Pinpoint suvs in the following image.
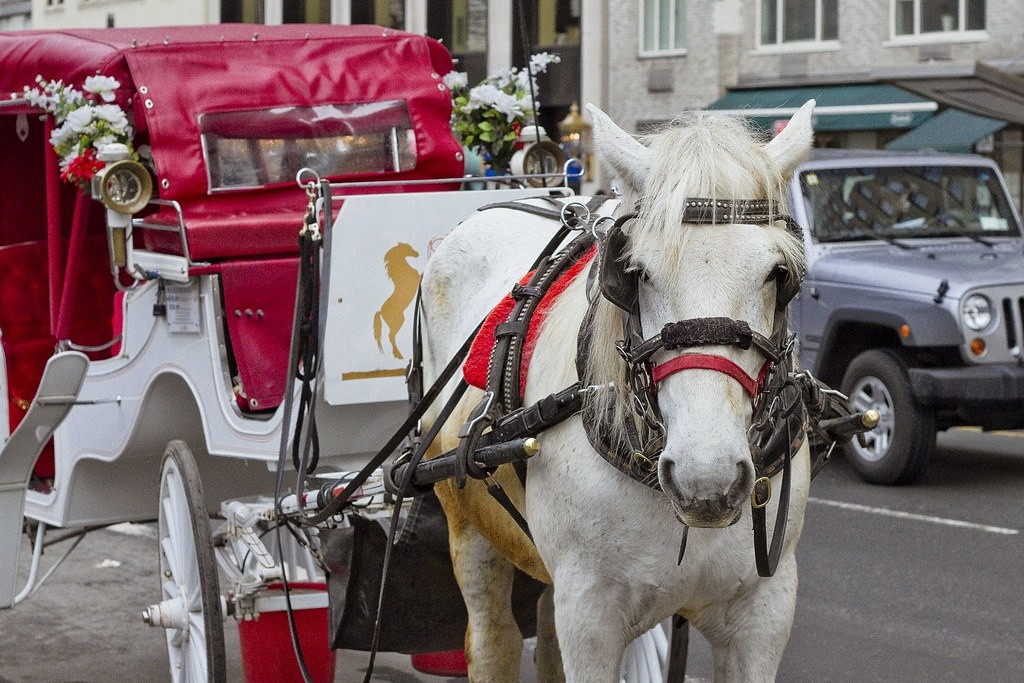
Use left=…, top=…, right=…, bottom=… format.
left=762, top=146, right=1024, bottom=488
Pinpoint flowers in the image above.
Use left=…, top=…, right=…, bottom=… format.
left=442, top=49, right=560, bottom=172
left=10, top=72, right=135, bottom=186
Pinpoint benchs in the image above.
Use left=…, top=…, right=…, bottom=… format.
left=0, top=234, right=115, bottom=436
left=142, top=189, right=448, bottom=411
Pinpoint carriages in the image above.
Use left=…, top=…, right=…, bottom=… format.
left=0, top=23, right=819, bottom=683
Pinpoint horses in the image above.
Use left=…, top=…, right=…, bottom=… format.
left=408, top=99, right=815, bottom=683
